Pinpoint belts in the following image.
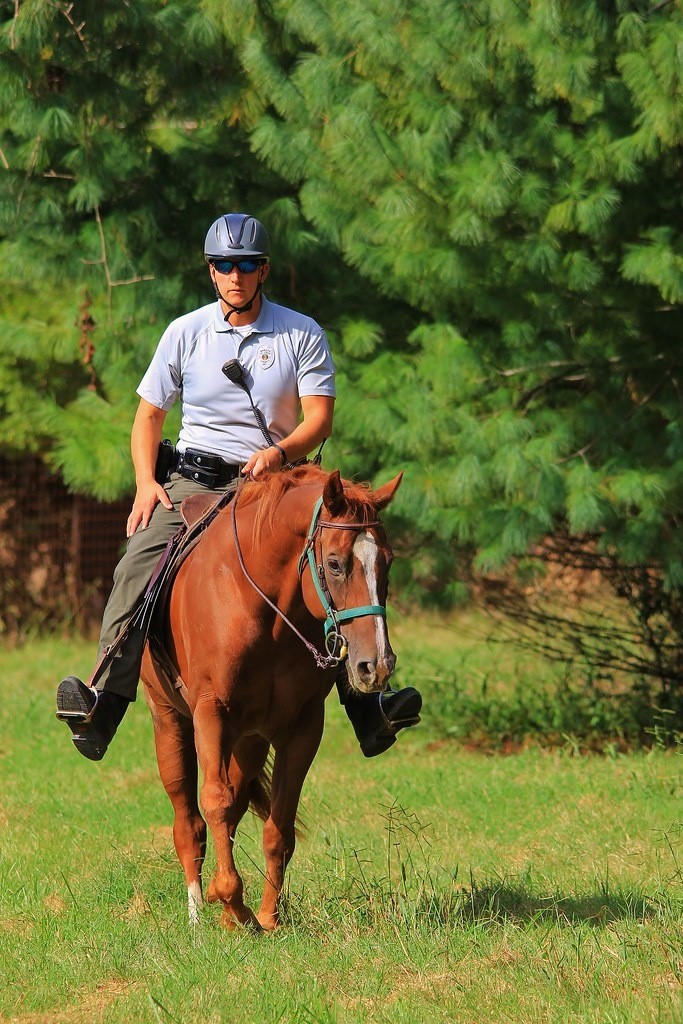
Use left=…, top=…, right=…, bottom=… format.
left=229, top=464, right=248, bottom=480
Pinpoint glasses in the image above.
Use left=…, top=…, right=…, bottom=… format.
left=208, top=258, right=267, bottom=275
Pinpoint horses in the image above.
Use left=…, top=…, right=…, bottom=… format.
left=140, top=461, right=406, bottom=938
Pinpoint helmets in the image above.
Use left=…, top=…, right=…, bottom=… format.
left=203, top=213, right=270, bottom=263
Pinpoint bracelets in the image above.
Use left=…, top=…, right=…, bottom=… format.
left=271, top=444, right=287, bottom=468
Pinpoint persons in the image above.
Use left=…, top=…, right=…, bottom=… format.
left=56, top=214, right=424, bottom=762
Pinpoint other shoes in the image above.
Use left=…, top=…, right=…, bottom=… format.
left=344, top=686, right=422, bottom=758
left=56, top=675, right=130, bottom=761
